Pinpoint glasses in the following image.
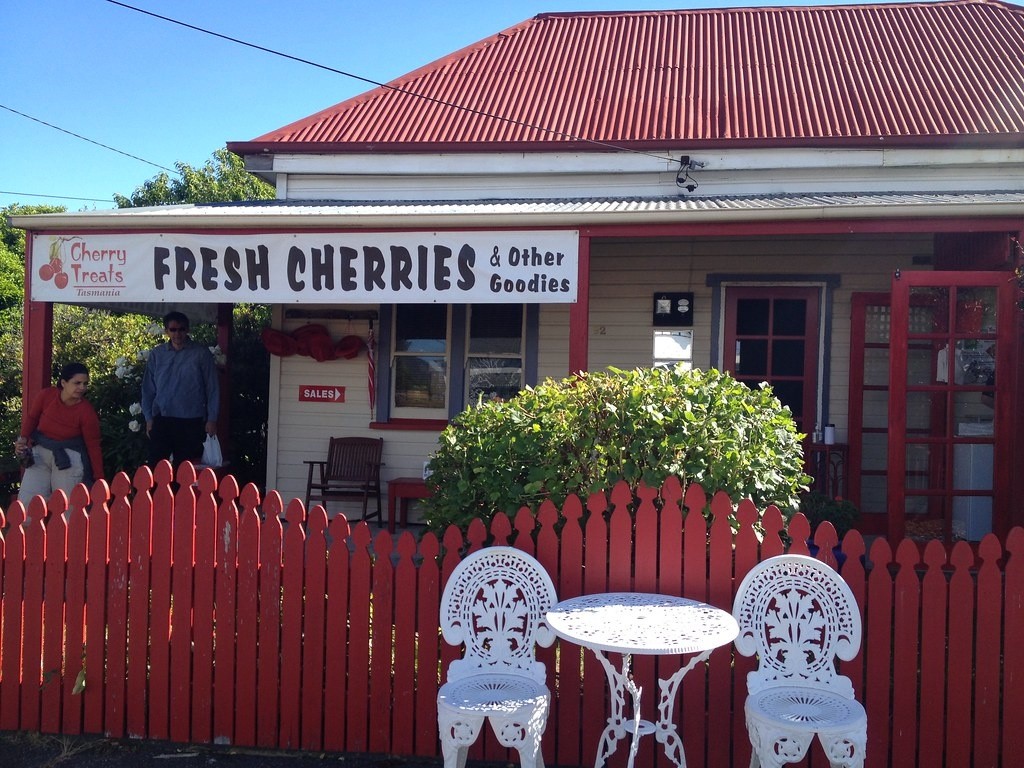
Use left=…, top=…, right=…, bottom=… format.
left=167, top=327, right=187, bottom=332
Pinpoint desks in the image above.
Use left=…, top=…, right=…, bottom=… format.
left=548, top=592, right=739, bottom=768
left=806, top=443, right=849, bottom=501
left=386, top=477, right=442, bottom=535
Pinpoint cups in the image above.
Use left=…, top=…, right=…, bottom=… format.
left=423, top=462, right=433, bottom=480
left=824, top=423, right=835, bottom=445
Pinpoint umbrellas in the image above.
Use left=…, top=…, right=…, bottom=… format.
left=366, top=311, right=375, bottom=421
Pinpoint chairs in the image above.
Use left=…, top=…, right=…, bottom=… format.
left=436, top=546, right=560, bottom=768
left=731, top=553, right=868, bottom=768
left=302, top=435, right=386, bottom=528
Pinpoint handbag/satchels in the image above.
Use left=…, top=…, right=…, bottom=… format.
left=201, top=432, right=223, bottom=468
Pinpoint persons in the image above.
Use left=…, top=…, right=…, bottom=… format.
left=15, top=363, right=107, bottom=529
left=140, top=311, right=219, bottom=494
